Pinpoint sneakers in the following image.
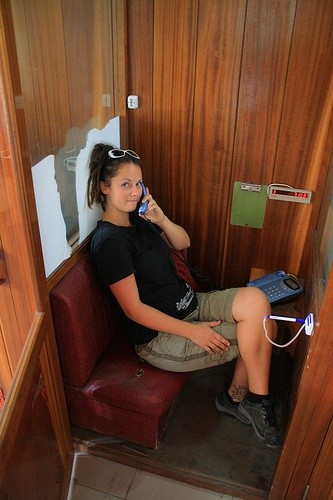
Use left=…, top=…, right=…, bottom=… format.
left=215, top=391, right=252, bottom=425
left=238, top=396, right=284, bottom=448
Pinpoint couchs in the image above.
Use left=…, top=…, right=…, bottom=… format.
left=49, top=256, right=192, bottom=450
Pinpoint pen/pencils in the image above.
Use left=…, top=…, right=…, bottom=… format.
left=267, top=315, right=305, bottom=323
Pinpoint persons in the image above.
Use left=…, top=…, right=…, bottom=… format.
left=87, top=143, right=287, bottom=449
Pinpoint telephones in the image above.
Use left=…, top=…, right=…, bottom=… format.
left=247, top=270, right=303, bottom=306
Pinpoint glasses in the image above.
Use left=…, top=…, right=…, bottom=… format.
left=103, top=149, right=140, bottom=162
